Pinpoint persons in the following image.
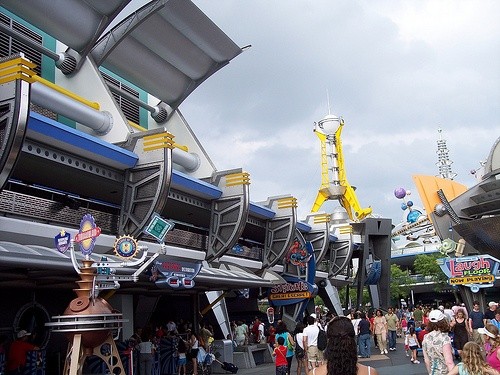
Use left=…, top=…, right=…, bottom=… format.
left=346, top=307, right=402, bottom=358
left=0, top=329, right=40, bottom=375
left=227, top=318, right=275, bottom=348
left=272, top=304, right=379, bottom=375
left=81, top=316, right=215, bottom=375
left=402, top=301, right=500, bottom=375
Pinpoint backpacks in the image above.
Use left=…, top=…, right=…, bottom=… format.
left=317, top=326, right=327, bottom=350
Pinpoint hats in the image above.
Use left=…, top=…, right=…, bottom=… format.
left=439, top=305, right=444, bottom=310
left=477, top=326, right=496, bottom=339
left=429, top=310, right=447, bottom=322
left=489, top=302, right=498, bottom=307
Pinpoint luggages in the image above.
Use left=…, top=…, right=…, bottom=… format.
left=214, top=359, right=238, bottom=374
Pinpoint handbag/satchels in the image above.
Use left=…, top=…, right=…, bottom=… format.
left=295, top=346, right=304, bottom=359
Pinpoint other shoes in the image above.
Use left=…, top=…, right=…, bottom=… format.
left=411, top=358, right=415, bottom=361
left=380, top=351, right=384, bottom=355
left=384, top=349, right=388, bottom=354
left=389, top=348, right=394, bottom=350
left=406, top=355, right=409, bottom=356
left=358, top=355, right=362, bottom=357
left=393, top=348, right=396, bottom=350
left=413, top=360, right=420, bottom=364
left=375, top=346, right=378, bottom=349
